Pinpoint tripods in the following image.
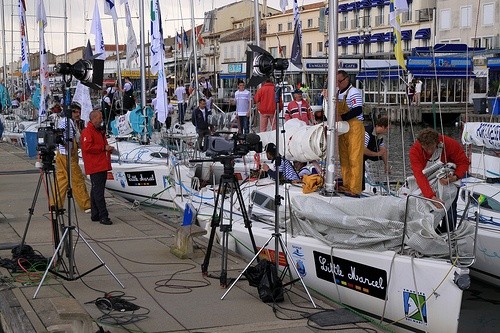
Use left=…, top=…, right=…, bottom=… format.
left=33, top=79, right=126, bottom=300
left=221, top=85, right=318, bottom=309
left=188, top=156, right=263, bottom=289
left=13, top=167, right=70, bottom=278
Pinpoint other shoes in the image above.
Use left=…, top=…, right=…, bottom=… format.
left=101, top=218, right=114, bottom=225
left=49, top=205, right=66, bottom=213
left=92, top=217, right=101, bottom=221
left=345, top=192, right=360, bottom=198
left=84, top=208, right=91, bottom=213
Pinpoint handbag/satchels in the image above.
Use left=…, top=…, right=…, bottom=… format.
left=203, top=88, right=213, bottom=98
left=302, top=175, right=323, bottom=193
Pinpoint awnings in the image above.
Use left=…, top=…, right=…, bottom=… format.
left=325, top=0, right=412, bottom=15
left=325, top=28, right=431, bottom=47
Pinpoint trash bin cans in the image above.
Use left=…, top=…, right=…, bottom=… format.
left=22, top=130, right=38, bottom=159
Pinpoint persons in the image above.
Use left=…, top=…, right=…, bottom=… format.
left=407, top=78, right=422, bottom=106
left=119, top=76, right=133, bottom=113
left=101, top=90, right=111, bottom=124
left=409, top=127, right=469, bottom=235
left=230, top=79, right=252, bottom=133
left=361, top=118, right=392, bottom=176
left=299, top=83, right=313, bottom=92
left=283, top=89, right=318, bottom=128
left=321, top=70, right=365, bottom=198
left=12, top=74, right=284, bottom=225
left=192, top=99, right=211, bottom=151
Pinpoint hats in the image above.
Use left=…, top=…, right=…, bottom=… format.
left=264, top=142, right=276, bottom=152
left=294, top=89, right=304, bottom=94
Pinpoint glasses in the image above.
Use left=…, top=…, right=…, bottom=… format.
left=337, top=76, right=348, bottom=84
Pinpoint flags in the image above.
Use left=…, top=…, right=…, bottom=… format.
left=16, top=0, right=303, bottom=125
left=390, top=0, right=410, bottom=72
